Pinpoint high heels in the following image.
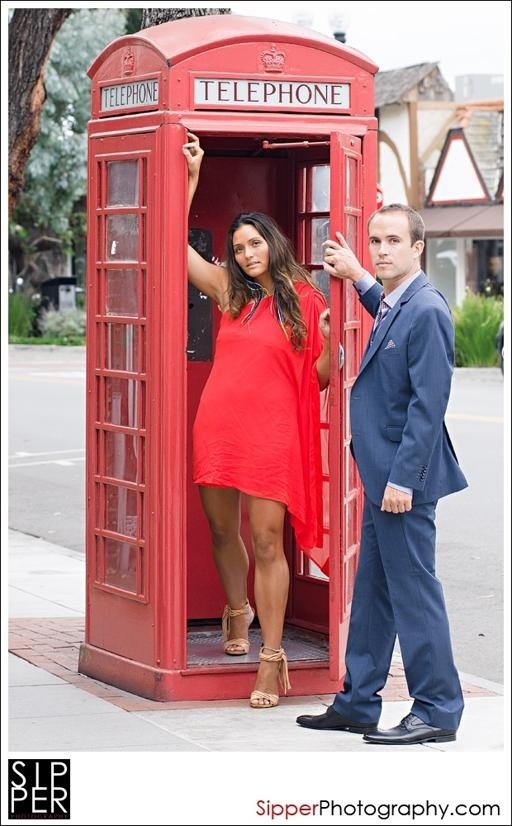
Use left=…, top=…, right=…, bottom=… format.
left=250, top=647, right=284, bottom=709
left=223, top=600, right=256, bottom=657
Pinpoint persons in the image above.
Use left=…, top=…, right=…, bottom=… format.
left=178, top=131, right=339, bottom=710
left=290, top=201, right=471, bottom=746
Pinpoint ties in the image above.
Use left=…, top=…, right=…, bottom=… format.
left=366, top=299, right=391, bottom=348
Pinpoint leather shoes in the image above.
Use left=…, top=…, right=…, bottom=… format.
left=362, top=710, right=458, bottom=746
left=295, top=702, right=378, bottom=734
